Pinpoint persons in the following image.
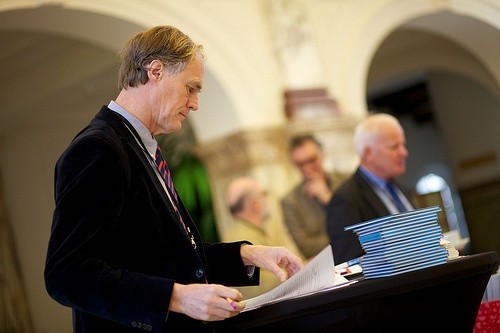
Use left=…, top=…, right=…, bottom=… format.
left=43, top=25, right=305, bottom=333
left=326, top=113, right=419, bottom=281
left=221, top=177, right=307, bottom=304
left=280, top=132, right=354, bottom=260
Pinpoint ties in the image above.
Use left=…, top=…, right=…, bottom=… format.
left=154, top=144, right=190, bottom=242
left=386, top=183, right=407, bottom=212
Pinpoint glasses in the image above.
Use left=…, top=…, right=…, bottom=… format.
left=296, top=153, right=321, bottom=167
